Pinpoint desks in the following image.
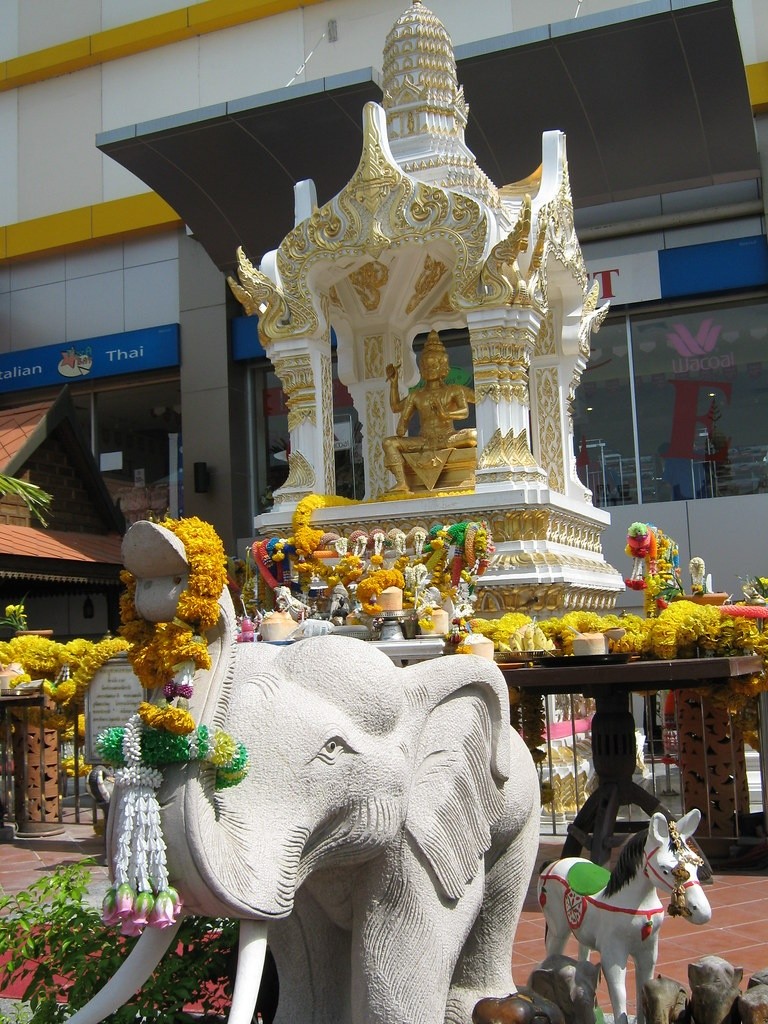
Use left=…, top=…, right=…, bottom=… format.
left=497, top=655, right=762, bottom=882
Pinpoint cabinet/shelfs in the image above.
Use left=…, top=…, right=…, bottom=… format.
left=577, top=427, right=768, bottom=508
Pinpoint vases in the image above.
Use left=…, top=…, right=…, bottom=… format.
left=15, top=629, right=53, bottom=640
left=671, top=591, right=727, bottom=607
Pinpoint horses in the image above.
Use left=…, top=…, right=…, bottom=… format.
left=537, top=808, right=712, bottom=1024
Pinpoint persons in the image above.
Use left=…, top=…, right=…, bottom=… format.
left=382, top=329, right=477, bottom=493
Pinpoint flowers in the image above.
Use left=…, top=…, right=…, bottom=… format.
left=735, top=574, right=768, bottom=606
left=101, top=886, right=183, bottom=936
left=0, top=593, right=28, bottom=631
left=651, top=544, right=705, bottom=603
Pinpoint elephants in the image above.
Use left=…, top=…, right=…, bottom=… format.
left=61, top=521, right=542, bottom=1024
